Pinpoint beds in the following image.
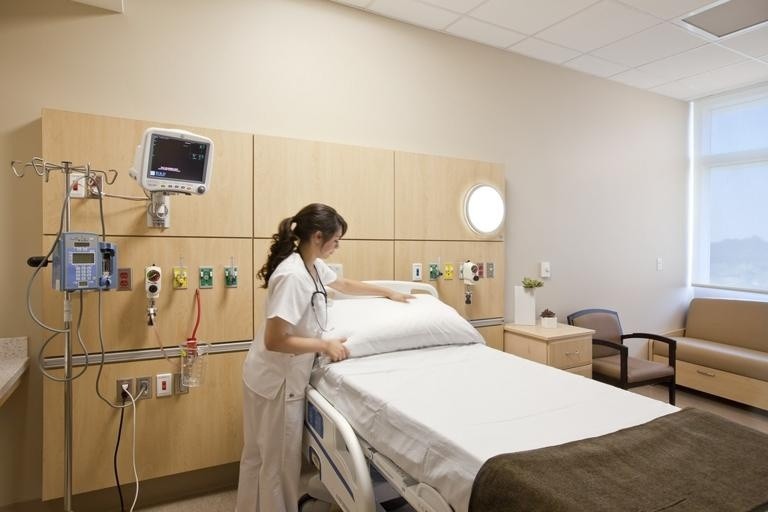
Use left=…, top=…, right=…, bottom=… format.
left=284, top=270, right=768, bottom=512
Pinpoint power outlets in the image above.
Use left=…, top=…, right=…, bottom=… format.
left=411, top=263, right=471, bottom=283
left=115, top=377, right=153, bottom=401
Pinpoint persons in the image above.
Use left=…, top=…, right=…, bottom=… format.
left=232, top=203, right=418, bottom=511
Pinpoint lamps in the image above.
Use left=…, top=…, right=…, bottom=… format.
left=463, top=182, right=506, bottom=238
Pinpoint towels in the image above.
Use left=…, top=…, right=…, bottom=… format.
left=465, top=406, right=768, bottom=512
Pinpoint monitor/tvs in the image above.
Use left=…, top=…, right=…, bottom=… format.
left=128, top=127, right=215, bottom=217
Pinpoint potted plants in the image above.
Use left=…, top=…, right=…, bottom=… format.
left=514, top=277, right=544, bottom=325
left=539, top=307, right=558, bottom=329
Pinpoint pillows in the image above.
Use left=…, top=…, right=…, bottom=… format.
left=301, top=290, right=490, bottom=372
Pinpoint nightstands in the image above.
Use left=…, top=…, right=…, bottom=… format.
left=503, top=317, right=595, bottom=382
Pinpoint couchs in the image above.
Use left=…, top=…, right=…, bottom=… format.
left=646, top=292, right=768, bottom=413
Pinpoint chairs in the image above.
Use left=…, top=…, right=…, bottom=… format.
left=565, top=304, right=677, bottom=410
